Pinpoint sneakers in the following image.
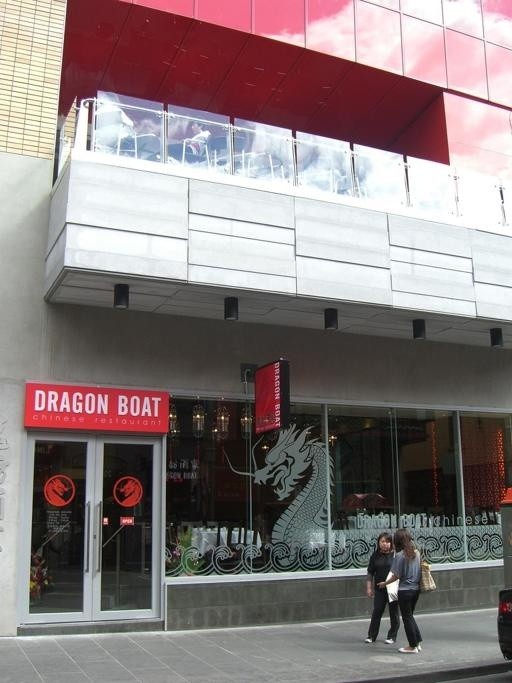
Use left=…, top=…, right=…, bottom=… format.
left=384, top=639, right=394, bottom=644
left=364, top=638, right=372, bottom=642
left=398, top=645, right=421, bottom=653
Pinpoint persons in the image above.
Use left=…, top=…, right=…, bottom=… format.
left=99, top=92, right=161, bottom=160
left=168, top=116, right=211, bottom=163
left=365, top=533, right=401, bottom=642
left=378, top=529, right=422, bottom=655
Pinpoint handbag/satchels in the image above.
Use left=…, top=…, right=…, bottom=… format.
left=385, top=571, right=400, bottom=603
left=420, top=561, right=436, bottom=592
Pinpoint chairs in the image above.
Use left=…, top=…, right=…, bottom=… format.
left=100, top=106, right=370, bottom=200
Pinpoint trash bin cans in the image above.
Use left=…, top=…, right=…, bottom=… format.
left=497, top=589, right=512, bottom=662
